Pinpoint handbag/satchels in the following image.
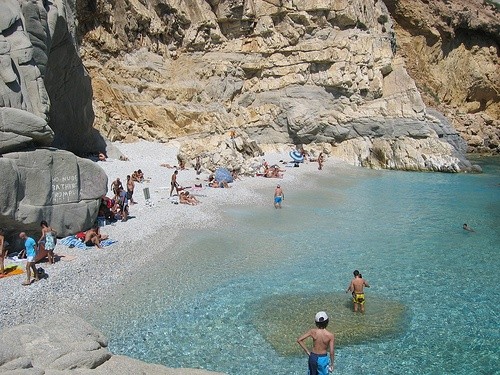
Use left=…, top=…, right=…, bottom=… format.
left=35, top=267, right=47, bottom=280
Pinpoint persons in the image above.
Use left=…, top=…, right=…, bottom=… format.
left=208, top=169, right=238, bottom=187
left=37, top=220, right=55, bottom=264
left=19, top=232, right=39, bottom=285
left=346, top=270, right=369, bottom=311
left=462, top=224, right=469, bottom=229
left=318, top=153, right=324, bottom=170
left=169, top=170, right=198, bottom=207
left=132, top=169, right=144, bottom=182
left=98, top=153, right=105, bottom=160
left=298, top=311, right=334, bottom=375
left=0, top=232, right=5, bottom=274
left=273, top=185, right=284, bottom=208
left=85, top=225, right=107, bottom=249
left=299, top=147, right=307, bottom=155
left=99, top=175, right=133, bottom=223
left=257, top=159, right=282, bottom=178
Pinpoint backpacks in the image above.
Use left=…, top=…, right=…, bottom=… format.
left=105, top=210, right=117, bottom=222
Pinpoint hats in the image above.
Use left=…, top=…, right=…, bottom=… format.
left=314, top=311, right=329, bottom=323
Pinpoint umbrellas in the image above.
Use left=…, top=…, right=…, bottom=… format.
left=289, top=151, right=304, bottom=162
